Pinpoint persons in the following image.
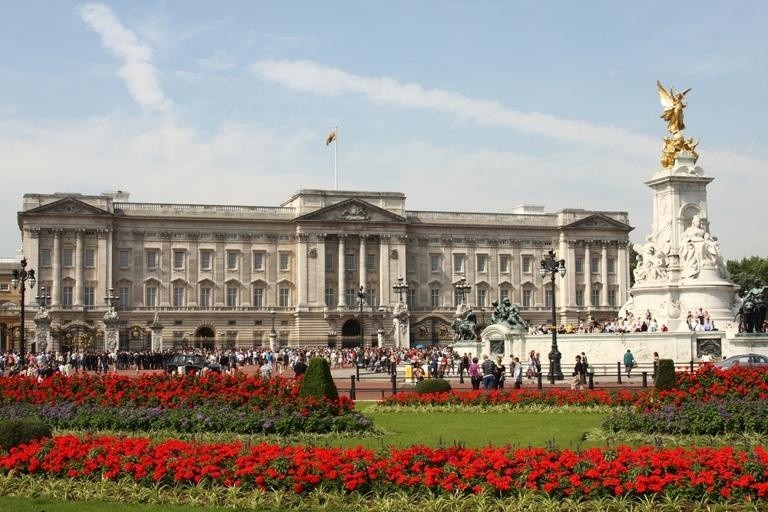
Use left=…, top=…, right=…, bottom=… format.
left=491, top=364, right=499, bottom=389
left=652, top=351, right=661, bottom=379
left=679, top=214, right=717, bottom=280
left=580, top=351, right=589, bottom=376
left=493, top=358, right=506, bottom=390
left=480, top=354, right=495, bottom=390
left=1, top=344, right=400, bottom=382
left=511, top=357, right=523, bottom=389
left=699, top=348, right=715, bottom=366
left=736, top=276, right=768, bottom=333
left=468, top=356, right=482, bottom=390
left=450, top=297, right=527, bottom=341
left=400, top=345, right=458, bottom=381
left=683, top=136, right=701, bottom=166
left=459, top=349, right=541, bottom=380
left=623, top=349, right=634, bottom=378
left=665, top=85, right=688, bottom=135
left=635, top=234, right=664, bottom=282
left=570, top=354, right=585, bottom=391
left=662, top=134, right=678, bottom=164
left=527, top=306, right=717, bottom=335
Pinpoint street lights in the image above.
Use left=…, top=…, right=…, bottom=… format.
left=540, top=248, right=566, bottom=380
left=356, top=285, right=367, bottom=368
left=11, top=256, right=36, bottom=368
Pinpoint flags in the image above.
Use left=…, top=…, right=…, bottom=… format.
left=325, top=129, right=337, bottom=147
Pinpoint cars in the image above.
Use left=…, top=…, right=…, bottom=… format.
left=717, top=354, right=768, bottom=369
left=167, top=353, right=221, bottom=378
